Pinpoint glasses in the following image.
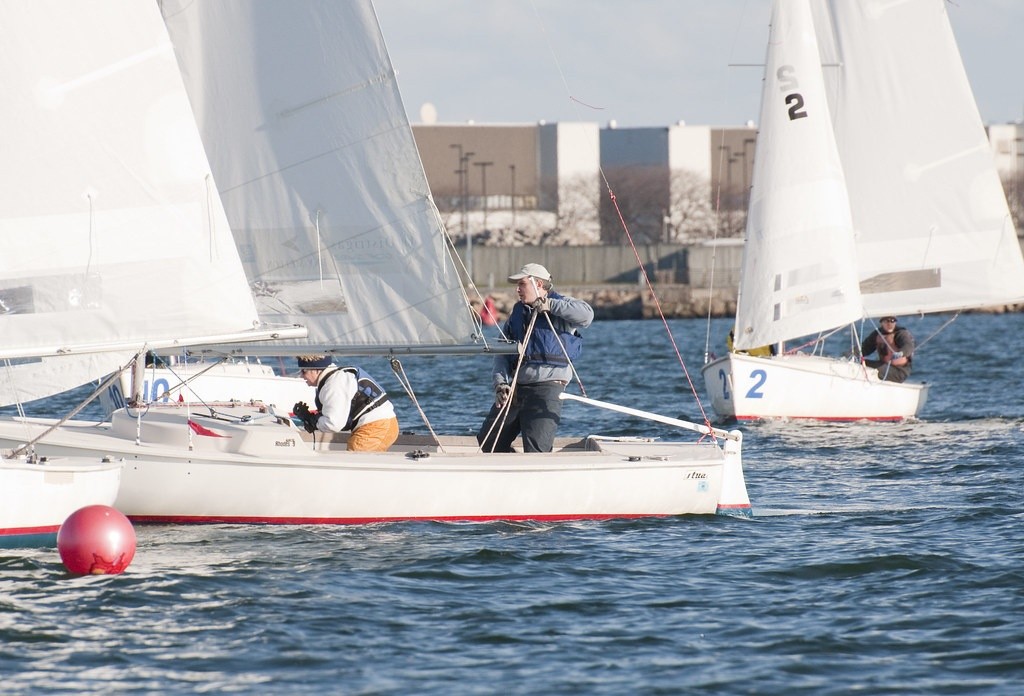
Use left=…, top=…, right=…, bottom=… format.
left=301, top=369, right=308, bottom=374
left=884, top=318, right=895, bottom=323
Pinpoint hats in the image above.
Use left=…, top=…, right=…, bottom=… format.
left=298, top=355, right=331, bottom=367
left=507, top=263, right=551, bottom=283
left=879, top=317, right=897, bottom=322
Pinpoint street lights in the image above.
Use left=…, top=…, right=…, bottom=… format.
left=475, top=162, right=494, bottom=230
left=736, top=139, right=755, bottom=211
left=718, top=145, right=738, bottom=215
left=450, top=144, right=475, bottom=233
left=506, top=164, right=516, bottom=225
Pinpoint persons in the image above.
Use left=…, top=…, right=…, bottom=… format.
left=290, top=356, right=402, bottom=453
left=727, top=317, right=777, bottom=360
left=480, top=263, right=594, bottom=453
left=837, top=315, right=914, bottom=385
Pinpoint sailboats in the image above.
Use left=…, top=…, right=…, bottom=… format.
left=700, top=1, right=1024, bottom=425
left=0, top=2, right=755, bottom=548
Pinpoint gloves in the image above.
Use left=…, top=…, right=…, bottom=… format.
left=293, top=401, right=323, bottom=433
left=532, top=297, right=552, bottom=313
left=495, top=384, right=511, bottom=408
